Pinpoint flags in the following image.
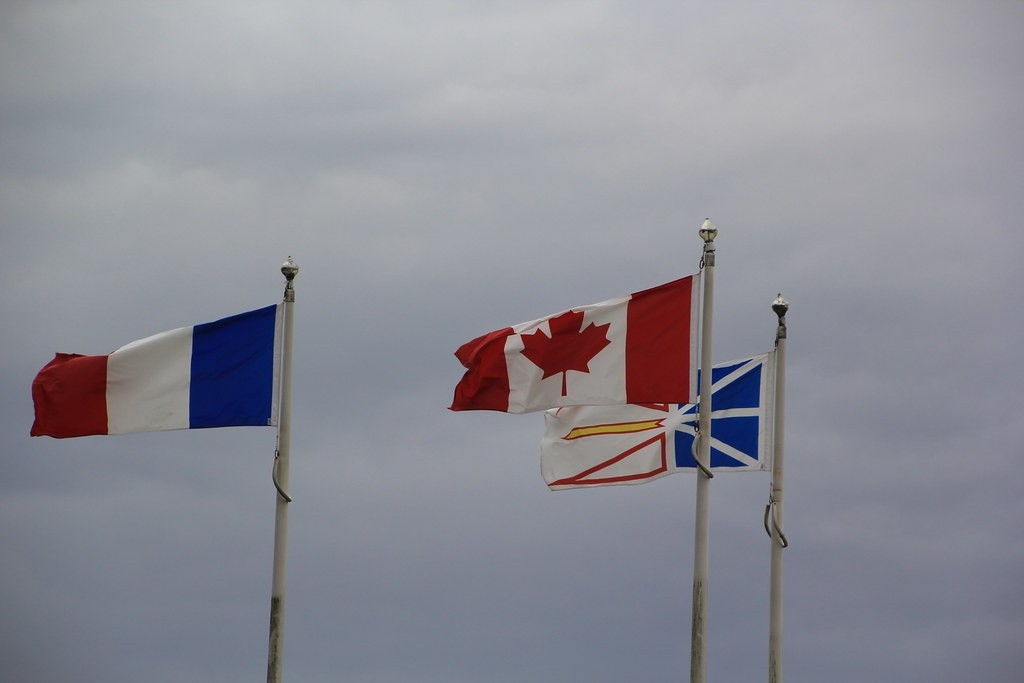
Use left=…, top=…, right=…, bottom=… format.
left=30, top=302, right=285, bottom=439
left=447, top=271, right=701, bottom=413
left=539, top=350, right=773, bottom=491
